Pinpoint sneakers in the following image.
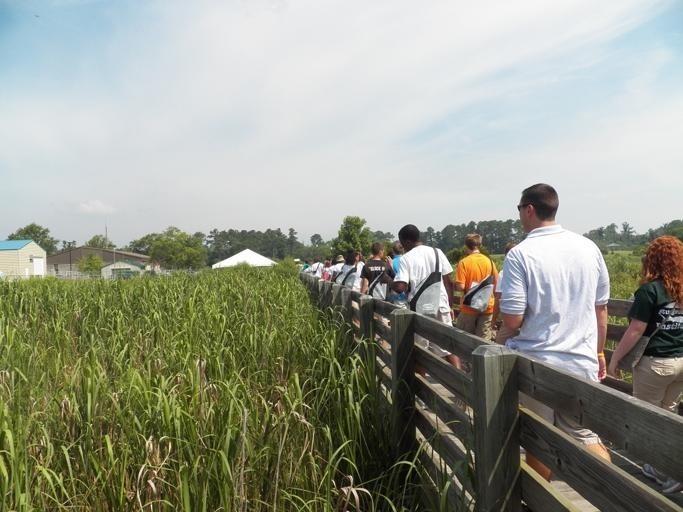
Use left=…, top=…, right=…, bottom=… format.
left=641, top=463, right=667, bottom=484
left=662, top=477, right=682, bottom=494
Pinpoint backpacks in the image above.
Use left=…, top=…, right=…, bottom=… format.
left=408, top=247, right=441, bottom=319
left=461, top=256, right=495, bottom=313
left=367, top=262, right=388, bottom=300
left=333, top=265, right=344, bottom=284
left=342, top=263, right=358, bottom=289
left=311, top=270, right=316, bottom=275
left=321, top=268, right=331, bottom=281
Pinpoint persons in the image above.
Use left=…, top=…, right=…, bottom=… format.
left=488, top=241, right=523, bottom=345
left=449, top=230, right=497, bottom=342
left=493, top=182, right=618, bottom=493
left=291, top=239, right=403, bottom=350
left=606, top=233, right=683, bottom=500
left=379, top=223, right=470, bottom=413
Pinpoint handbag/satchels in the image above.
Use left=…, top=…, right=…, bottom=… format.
left=617, top=335, right=651, bottom=372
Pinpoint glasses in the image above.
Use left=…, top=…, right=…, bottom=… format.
left=517, top=202, right=535, bottom=211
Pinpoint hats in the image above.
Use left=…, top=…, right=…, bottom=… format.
left=335, top=255, right=345, bottom=262
